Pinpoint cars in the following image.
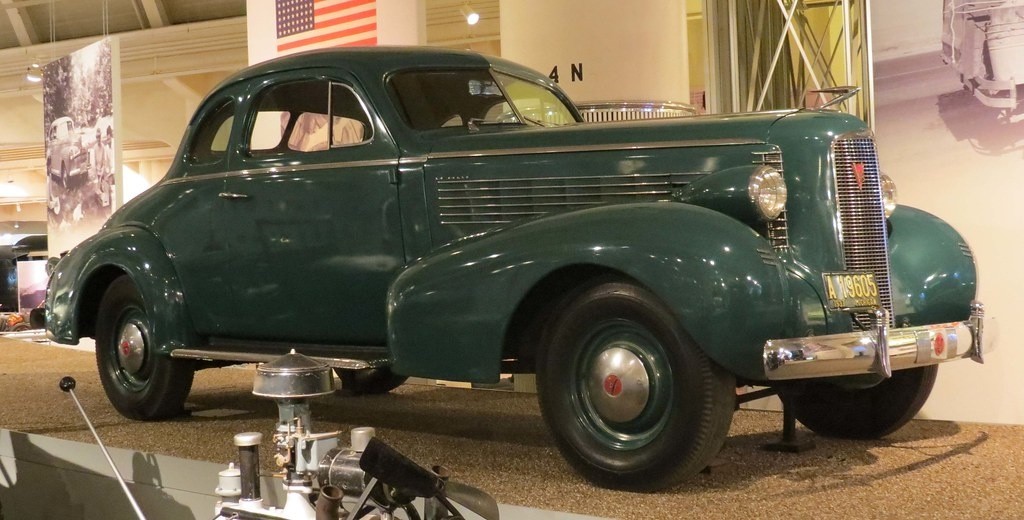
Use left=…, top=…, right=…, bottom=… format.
left=29, top=46, right=984, bottom=494
left=46, top=116, right=93, bottom=189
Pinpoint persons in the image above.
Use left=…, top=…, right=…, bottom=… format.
left=91, top=126, right=115, bottom=192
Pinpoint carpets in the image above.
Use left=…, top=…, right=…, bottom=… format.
left=0, top=336, right=1024, bottom=520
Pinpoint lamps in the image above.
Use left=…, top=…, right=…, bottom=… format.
left=460, top=0, right=479, bottom=25
left=26, top=58, right=42, bottom=83
